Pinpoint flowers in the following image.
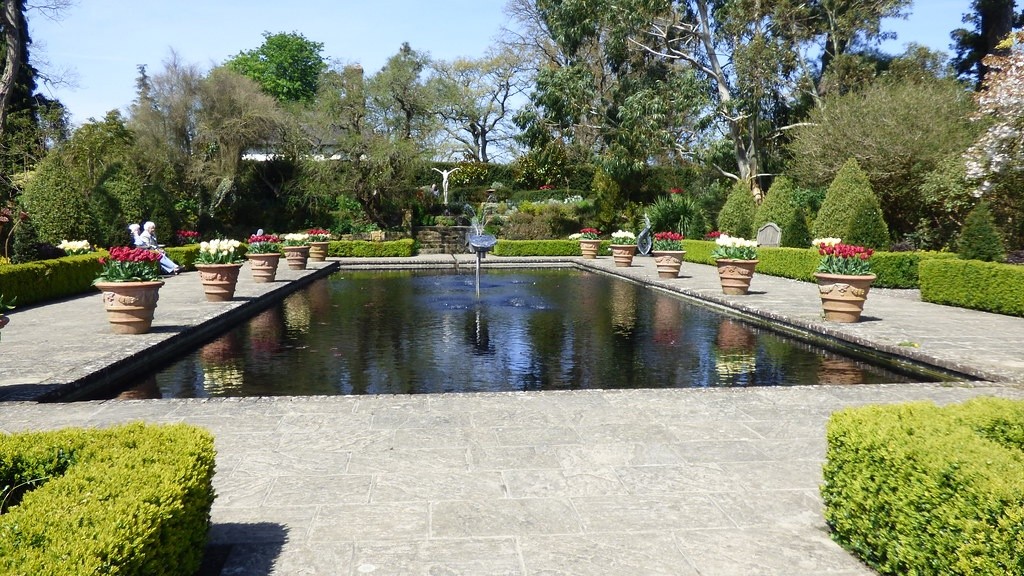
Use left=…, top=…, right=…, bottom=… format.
left=610, top=229, right=639, bottom=245
left=251, top=329, right=284, bottom=353
left=706, top=234, right=762, bottom=261
left=652, top=233, right=685, bottom=251
left=611, top=311, right=637, bottom=335
left=89, top=246, right=166, bottom=286
left=284, top=233, right=310, bottom=247
left=811, top=238, right=876, bottom=276
left=580, top=228, right=602, bottom=240
left=652, top=328, right=681, bottom=349
left=307, top=229, right=330, bottom=242
left=203, top=356, right=246, bottom=396
left=710, top=347, right=757, bottom=381
left=246, top=234, right=281, bottom=254
left=191, top=238, right=244, bottom=264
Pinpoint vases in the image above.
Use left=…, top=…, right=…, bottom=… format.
left=94, top=280, right=165, bottom=334
left=201, top=332, right=234, bottom=366
left=245, top=253, right=282, bottom=282
left=813, top=272, right=878, bottom=323
left=283, top=246, right=311, bottom=270
left=655, top=295, right=681, bottom=329
left=308, top=242, right=330, bottom=261
left=610, top=244, right=637, bottom=268
left=815, top=356, right=863, bottom=386
left=610, top=278, right=637, bottom=312
left=195, top=264, right=243, bottom=302
left=715, top=316, right=756, bottom=352
left=652, top=250, right=687, bottom=279
left=250, top=306, right=280, bottom=329
left=579, top=240, right=602, bottom=259
left=715, top=259, right=759, bottom=295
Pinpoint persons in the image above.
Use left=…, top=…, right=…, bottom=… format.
left=434, top=168, right=460, bottom=202
left=129, top=224, right=148, bottom=246
left=140, top=222, right=185, bottom=275
